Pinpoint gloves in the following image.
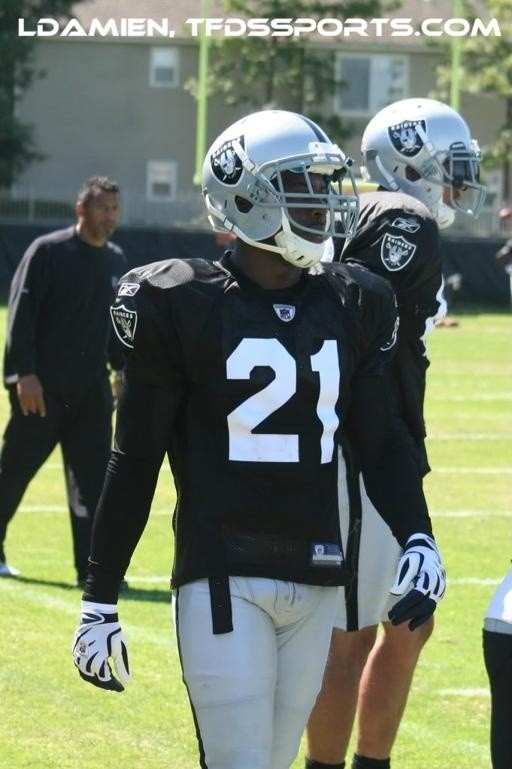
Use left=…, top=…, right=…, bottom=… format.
left=387, top=533, right=446, bottom=632
left=72, top=600, right=130, bottom=692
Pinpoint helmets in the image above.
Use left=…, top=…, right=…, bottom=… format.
left=202, top=108, right=359, bottom=242
left=361, top=97, right=487, bottom=219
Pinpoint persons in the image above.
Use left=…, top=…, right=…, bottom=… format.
left=436, top=246, right=463, bottom=329
left=70, top=105, right=452, bottom=768
left=302, top=93, right=488, bottom=769
left=494, top=205, right=511, bottom=298
left=0, top=174, right=132, bottom=595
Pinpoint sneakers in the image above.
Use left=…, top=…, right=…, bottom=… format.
left=76, top=562, right=130, bottom=592
left=0, top=561, right=21, bottom=575
